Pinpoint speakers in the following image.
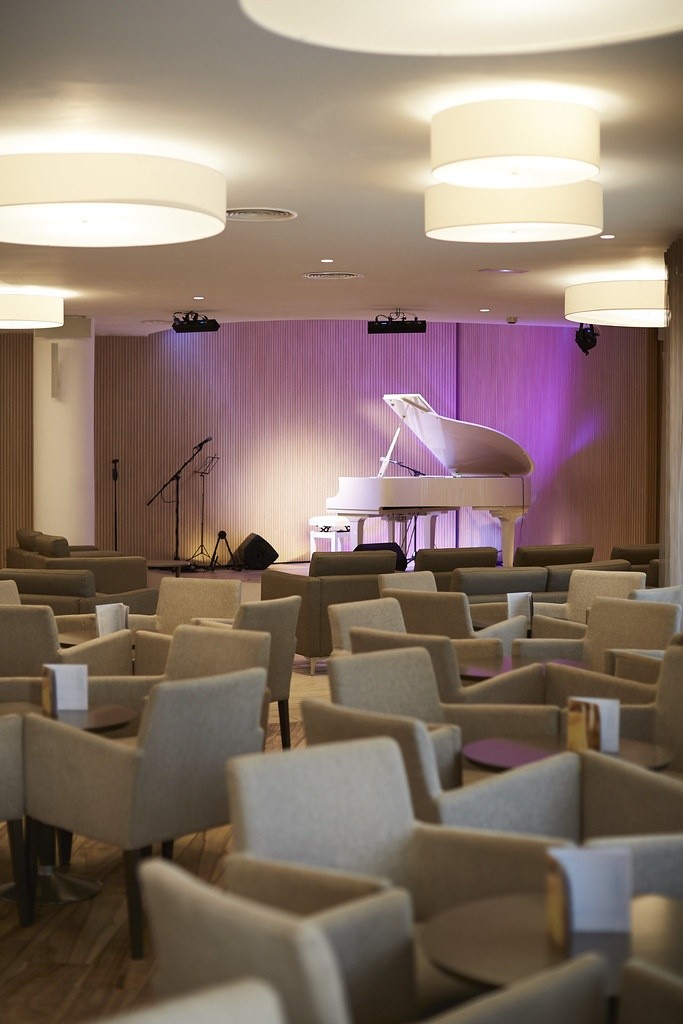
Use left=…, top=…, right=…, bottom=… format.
left=238, top=533, right=279, bottom=569
left=353, top=542, right=408, bottom=572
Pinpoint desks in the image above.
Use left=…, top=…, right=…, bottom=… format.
left=472, top=617, right=569, bottom=631
left=57, top=630, right=158, bottom=650
left=461, top=735, right=674, bottom=773
left=0, top=701, right=139, bottom=907
left=420, top=894, right=683, bottom=1024
left=458, top=656, right=590, bottom=682
left=146, top=560, right=190, bottom=578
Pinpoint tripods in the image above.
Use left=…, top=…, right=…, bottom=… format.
left=186, top=454, right=223, bottom=567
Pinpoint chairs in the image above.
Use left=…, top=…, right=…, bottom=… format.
left=0, top=529, right=683, bottom=1024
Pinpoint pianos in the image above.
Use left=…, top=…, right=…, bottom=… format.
left=326, top=390, right=536, bottom=567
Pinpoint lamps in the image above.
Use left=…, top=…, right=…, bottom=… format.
left=564, top=280, right=670, bottom=328
left=0, top=154, right=227, bottom=247
left=424, top=182, right=604, bottom=243
left=429, top=98, right=601, bottom=190
left=0, top=292, right=63, bottom=330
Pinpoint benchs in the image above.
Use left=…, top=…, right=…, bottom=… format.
left=308, top=517, right=352, bottom=560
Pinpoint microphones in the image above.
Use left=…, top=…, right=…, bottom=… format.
left=193, top=436, right=213, bottom=449
left=379, top=456, right=399, bottom=464
